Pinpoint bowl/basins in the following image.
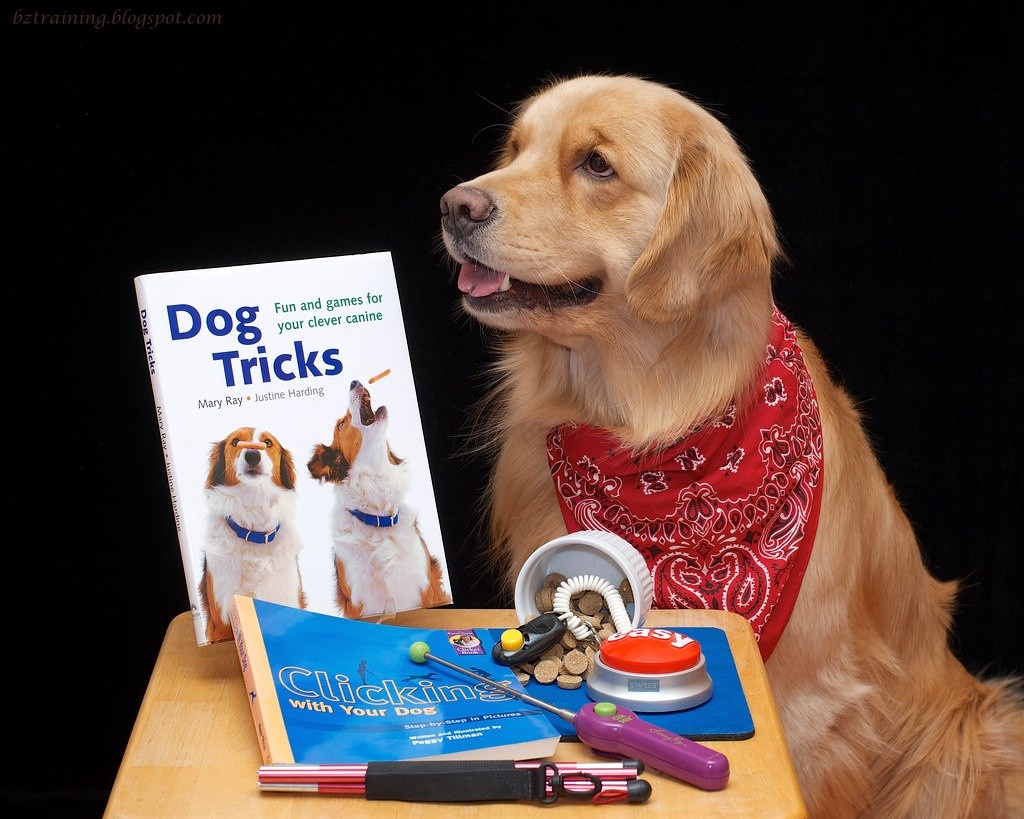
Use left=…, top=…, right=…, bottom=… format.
left=514, top=530, right=653, bottom=641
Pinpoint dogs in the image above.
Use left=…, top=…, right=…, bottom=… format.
left=438, top=73, right=1024, bottom=819
left=306, top=379, right=452, bottom=620
left=197, top=424, right=308, bottom=644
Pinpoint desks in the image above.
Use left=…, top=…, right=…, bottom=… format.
left=102, top=608, right=810, bottom=819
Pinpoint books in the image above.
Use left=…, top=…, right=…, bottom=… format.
left=229, top=593, right=561, bottom=766
left=135, top=250, right=455, bottom=648
